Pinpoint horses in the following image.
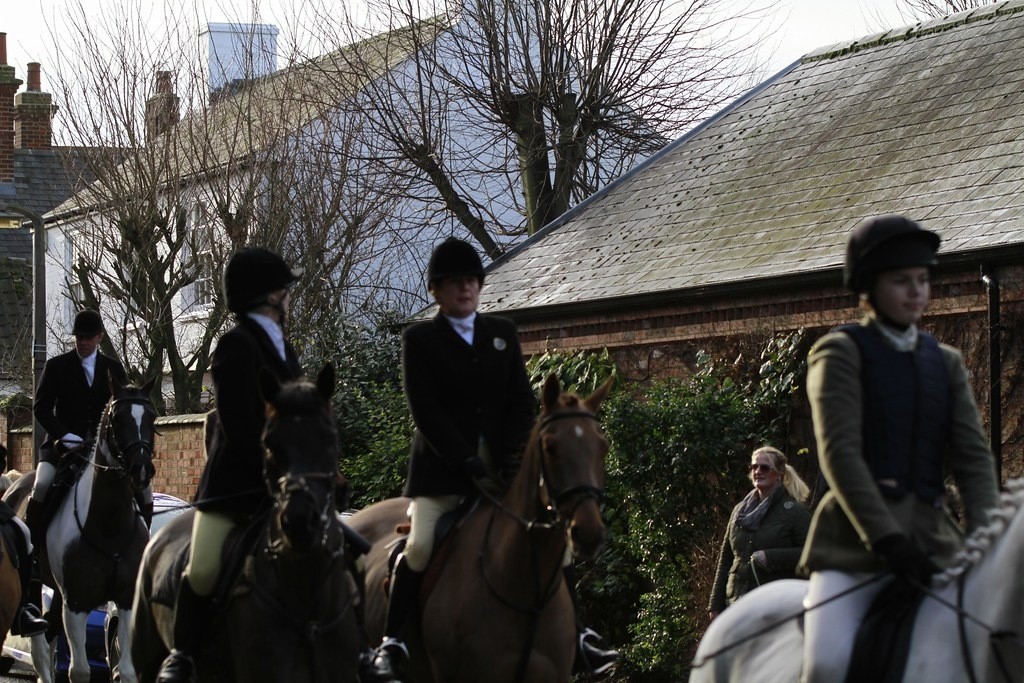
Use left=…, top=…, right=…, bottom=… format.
left=336, top=373, right=617, bottom=683
left=0, top=370, right=379, bottom=683
left=690, top=468, right=1024, bottom=683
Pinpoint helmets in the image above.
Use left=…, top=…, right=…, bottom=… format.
left=844, top=216, right=940, bottom=292
left=69, top=307, right=102, bottom=337
left=222, top=247, right=303, bottom=312
left=426, top=238, right=484, bottom=292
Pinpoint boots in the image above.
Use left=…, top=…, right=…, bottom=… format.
left=25, top=497, right=54, bottom=578
left=373, top=552, right=426, bottom=678
left=156, top=572, right=217, bottom=683
left=564, top=564, right=620, bottom=676
left=354, top=566, right=375, bottom=665
left=16, top=547, right=49, bottom=636
left=137, top=501, right=153, bottom=530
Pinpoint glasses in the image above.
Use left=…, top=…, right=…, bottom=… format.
left=748, top=464, right=771, bottom=472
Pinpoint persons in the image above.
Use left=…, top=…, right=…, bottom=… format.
left=708, top=446, right=811, bottom=621
left=370, top=236, right=614, bottom=683
left=157, top=247, right=304, bottom=683
left=26, top=309, right=153, bottom=617
left=0, top=498, right=49, bottom=635
left=798, top=215, right=1000, bottom=683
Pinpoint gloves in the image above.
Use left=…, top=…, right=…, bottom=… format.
left=459, top=455, right=485, bottom=479
left=61, top=433, right=83, bottom=450
left=873, top=535, right=941, bottom=583
